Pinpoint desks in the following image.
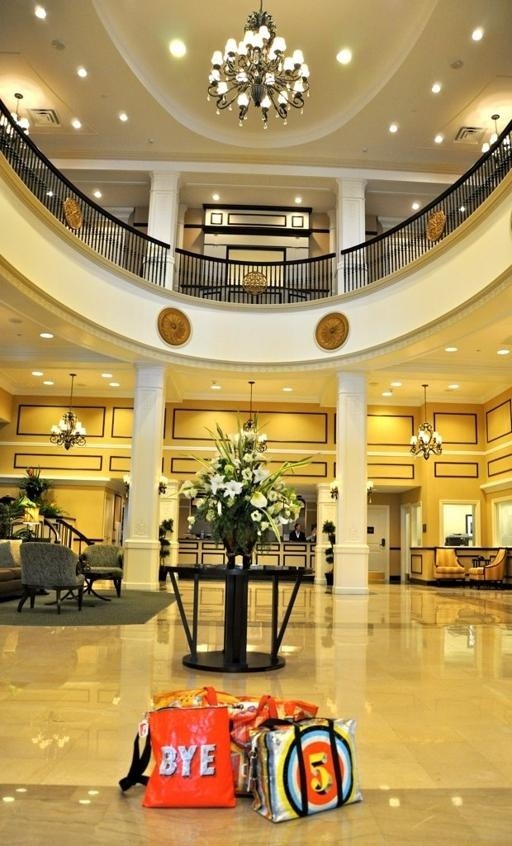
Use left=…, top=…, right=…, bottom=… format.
left=45, top=571, right=113, bottom=605
left=164, top=564, right=314, bottom=672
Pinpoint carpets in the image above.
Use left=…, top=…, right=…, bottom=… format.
left=0, top=590, right=184, bottom=626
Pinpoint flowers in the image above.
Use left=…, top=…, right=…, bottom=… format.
left=178, top=410, right=313, bottom=548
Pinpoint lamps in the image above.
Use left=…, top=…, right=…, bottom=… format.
left=207, top=0, right=310, bottom=129
left=482, top=115, right=512, bottom=165
left=49, top=374, right=86, bottom=449
left=159, top=477, right=167, bottom=495
left=123, top=474, right=130, bottom=499
left=243, top=381, right=267, bottom=454
left=1, top=93, right=30, bottom=150
left=410, top=385, right=442, bottom=460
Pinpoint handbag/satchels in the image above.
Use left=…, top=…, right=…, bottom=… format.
left=120, top=687, right=363, bottom=823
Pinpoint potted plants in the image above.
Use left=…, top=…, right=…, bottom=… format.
left=322, top=520, right=335, bottom=586
left=159, top=518, right=174, bottom=583
left=39, top=491, right=60, bottom=525
left=17, top=464, right=52, bottom=502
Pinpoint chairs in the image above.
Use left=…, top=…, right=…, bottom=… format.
left=467, top=549, right=507, bottom=590
left=81, top=545, right=123, bottom=597
left=17, top=542, right=84, bottom=614
left=433, top=547, right=466, bottom=588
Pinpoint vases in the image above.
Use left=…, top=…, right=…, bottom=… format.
left=218, top=527, right=258, bottom=569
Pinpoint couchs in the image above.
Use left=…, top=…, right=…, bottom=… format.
left=0, top=539, right=25, bottom=598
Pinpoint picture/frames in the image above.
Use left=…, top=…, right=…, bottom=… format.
left=466, top=515, right=472, bottom=537
